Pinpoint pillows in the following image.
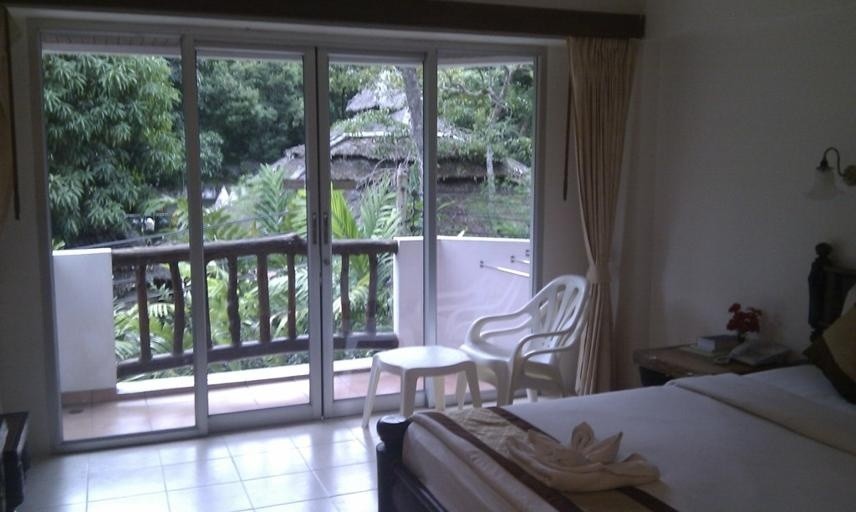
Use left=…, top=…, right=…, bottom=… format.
left=802, top=303, right=856, bottom=407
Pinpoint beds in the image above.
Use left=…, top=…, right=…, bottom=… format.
left=377, top=241, right=856, bottom=512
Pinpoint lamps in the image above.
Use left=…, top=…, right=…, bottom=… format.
left=803, top=147, right=856, bottom=197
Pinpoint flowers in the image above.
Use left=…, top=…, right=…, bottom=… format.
left=727, top=303, right=763, bottom=333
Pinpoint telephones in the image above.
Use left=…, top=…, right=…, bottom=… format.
left=730, top=340, right=791, bottom=367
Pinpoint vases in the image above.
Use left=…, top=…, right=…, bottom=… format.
left=737, top=329, right=749, bottom=344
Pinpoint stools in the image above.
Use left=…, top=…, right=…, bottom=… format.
left=363, top=344, right=481, bottom=431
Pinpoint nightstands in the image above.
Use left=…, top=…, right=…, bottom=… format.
left=633, top=339, right=807, bottom=386
